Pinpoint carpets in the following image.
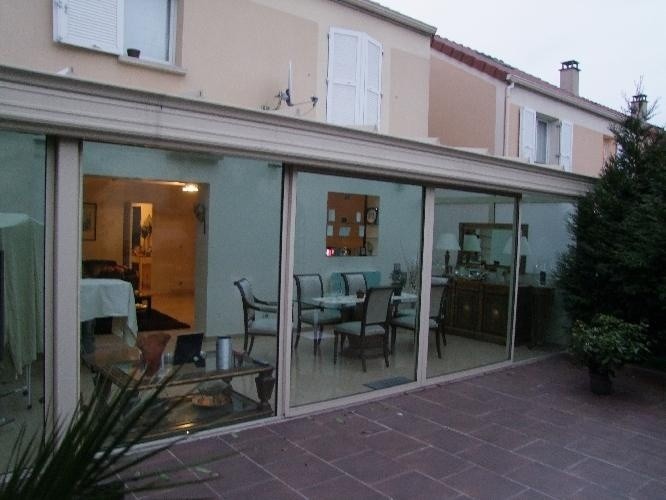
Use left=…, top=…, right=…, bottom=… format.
left=361, top=377, right=415, bottom=390
left=339, top=344, right=389, bottom=361
left=96, top=307, right=190, bottom=334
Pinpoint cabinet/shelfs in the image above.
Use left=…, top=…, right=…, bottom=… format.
left=448, top=281, right=536, bottom=348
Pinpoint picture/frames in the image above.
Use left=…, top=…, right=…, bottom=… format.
left=82, top=203, right=96, bottom=241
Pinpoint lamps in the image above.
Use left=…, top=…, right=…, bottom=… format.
left=519, top=236, right=529, bottom=256
left=435, top=233, right=462, bottom=275
left=462, top=234, right=481, bottom=271
left=502, top=235, right=515, bottom=256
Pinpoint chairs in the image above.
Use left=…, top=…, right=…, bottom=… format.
left=232, top=271, right=451, bottom=373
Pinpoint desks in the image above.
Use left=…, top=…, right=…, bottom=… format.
left=81, top=277, right=138, bottom=337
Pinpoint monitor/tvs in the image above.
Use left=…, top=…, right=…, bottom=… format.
left=327, top=246, right=337, bottom=256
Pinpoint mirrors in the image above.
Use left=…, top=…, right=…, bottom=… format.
left=458, top=222, right=529, bottom=275
